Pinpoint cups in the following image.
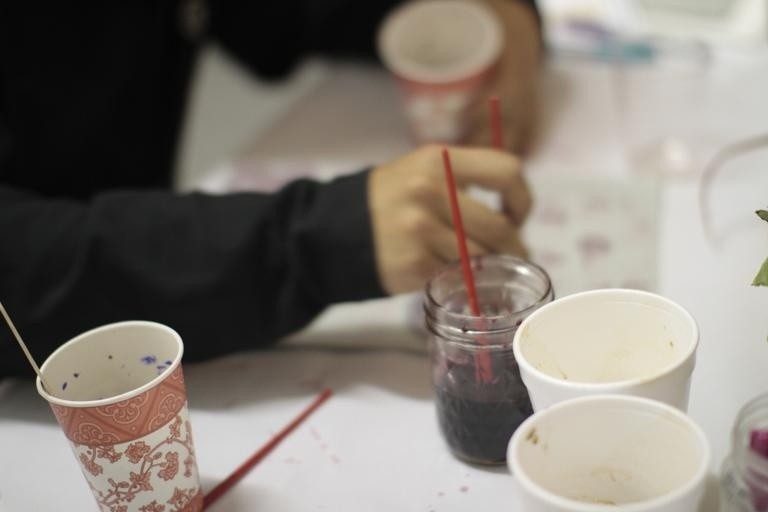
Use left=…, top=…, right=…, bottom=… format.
left=424, top=256, right=557, bottom=468
left=510, top=287, right=700, bottom=416
left=35, top=319, right=204, bottom=512
left=378, top=0, right=509, bottom=151
left=509, top=394, right=710, bottom=508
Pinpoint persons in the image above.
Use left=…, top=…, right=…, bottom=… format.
left=0, top=1, right=533, bottom=379
